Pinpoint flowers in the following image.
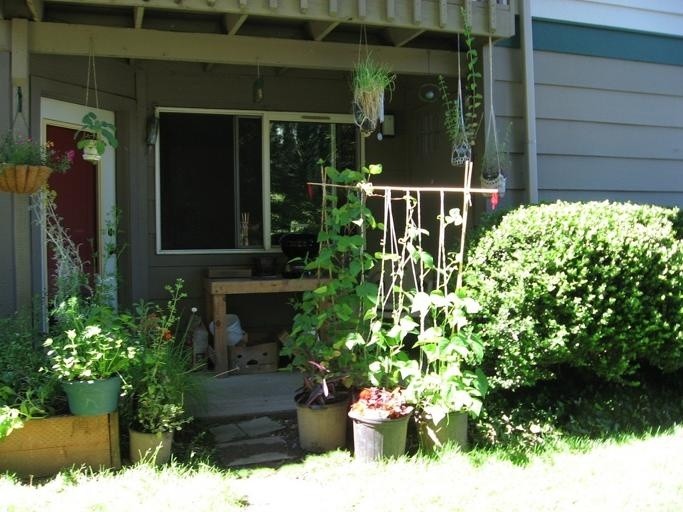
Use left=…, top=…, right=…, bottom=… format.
left=350, top=386, right=414, bottom=422
left=0, top=130, right=76, bottom=172
left=127, top=279, right=209, bottom=433
left=299, top=360, right=346, bottom=407
left=42, top=296, right=141, bottom=398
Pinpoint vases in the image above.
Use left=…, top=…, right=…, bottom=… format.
left=60, top=376, right=120, bottom=417
left=348, top=408, right=413, bottom=462
left=0, top=163, right=53, bottom=194
left=127, top=422, right=175, bottom=467
left=1, top=410, right=123, bottom=482
left=296, top=395, right=351, bottom=453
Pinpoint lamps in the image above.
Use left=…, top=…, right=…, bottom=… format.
left=418, top=47, right=440, bottom=104
left=253, top=55, right=265, bottom=104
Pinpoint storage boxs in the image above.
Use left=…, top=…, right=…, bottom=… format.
left=229, top=330, right=279, bottom=374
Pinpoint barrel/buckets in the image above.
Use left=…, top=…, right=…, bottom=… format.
left=208, top=312, right=244, bottom=346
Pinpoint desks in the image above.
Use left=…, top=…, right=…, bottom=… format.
left=206, top=277, right=340, bottom=378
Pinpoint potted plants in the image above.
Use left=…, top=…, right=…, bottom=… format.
left=73, top=112, right=119, bottom=161
left=348, top=47, right=397, bottom=133
left=414, top=291, right=492, bottom=456
left=438, top=4, right=489, bottom=167
left=0, top=293, right=121, bottom=444
left=480, top=120, right=513, bottom=199
left=274, top=218, right=320, bottom=276
